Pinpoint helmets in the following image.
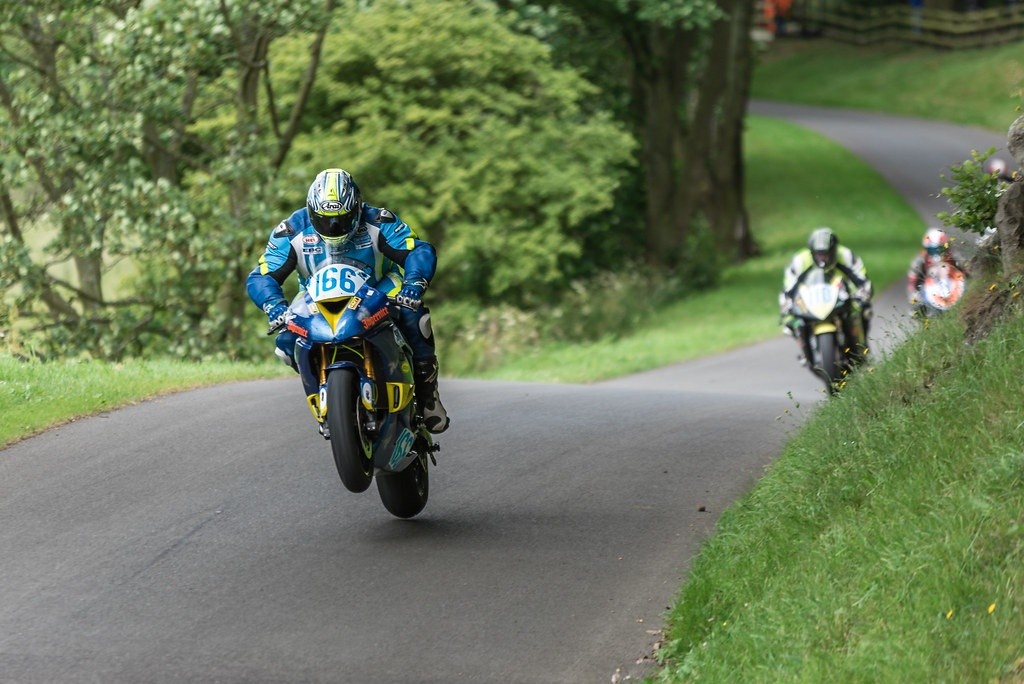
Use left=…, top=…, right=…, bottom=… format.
left=987, top=158, right=1008, bottom=177
left=807, top=228, right=838, bottom=269
left=307, top=168, right=362, bottom=249
left=922, top=228, right=953, bottom=265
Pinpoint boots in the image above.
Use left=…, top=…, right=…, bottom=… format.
left=412, top=356, right=449, bottom=435
left=319, top=420, right=331, bottom=439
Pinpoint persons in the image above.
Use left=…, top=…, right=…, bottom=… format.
left=907, top=228, right=975, bottom=298
left=246, top=168, right=450, bottom=434
left=777, top=228, right=873, bottom=363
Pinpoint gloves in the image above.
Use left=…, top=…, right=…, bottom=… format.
left=851, top=290, right=870, bottom=308
left=262, top=297, right=289, bottom=327
left=781, top=314, right=797, bottom=330
left=394, top=277, right=428, bottom=311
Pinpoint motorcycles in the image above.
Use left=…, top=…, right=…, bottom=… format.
left=908, top=266, right=965, bottom=319
left=779, top=265, right=871, bottom=396
left=267, top=237, right=437, bottom=520
